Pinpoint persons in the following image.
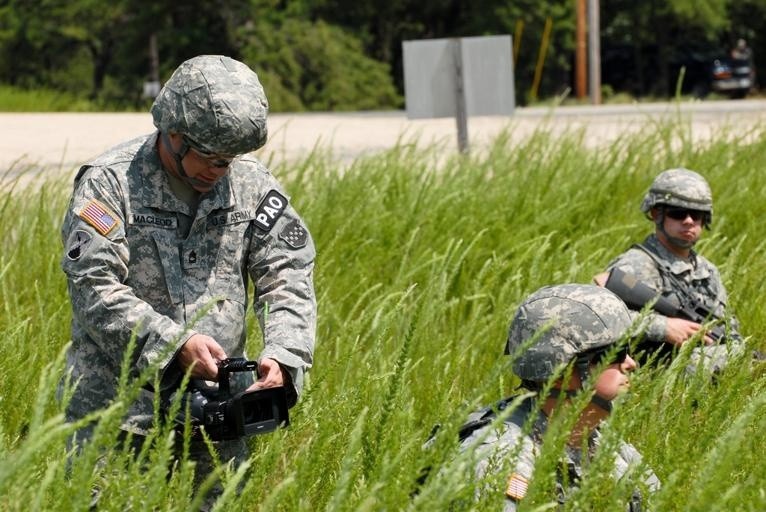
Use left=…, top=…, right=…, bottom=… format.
left=410, top=281, right=662, bottom=512
left=588, top=167, right=766, bottom=380
left=54, top=52, right=320, bottom=511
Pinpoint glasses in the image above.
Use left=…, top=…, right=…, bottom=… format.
left=591, top=344, right=629, bottom=364
left=656, top=205, right=705, bottom=221
left=182, top=133, right=235, bottom=168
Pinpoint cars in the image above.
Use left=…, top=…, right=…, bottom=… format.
left=673, top=49, right=753, bottom=98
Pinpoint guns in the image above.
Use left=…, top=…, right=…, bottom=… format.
left=605, top=265, right=766, bottom=364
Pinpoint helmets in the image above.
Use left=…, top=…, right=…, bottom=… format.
left=508, top=283, right=634, bottom=382
left=641, top=168, right=713, bottom=223
left=150, top=54, right=270, bottom=157
